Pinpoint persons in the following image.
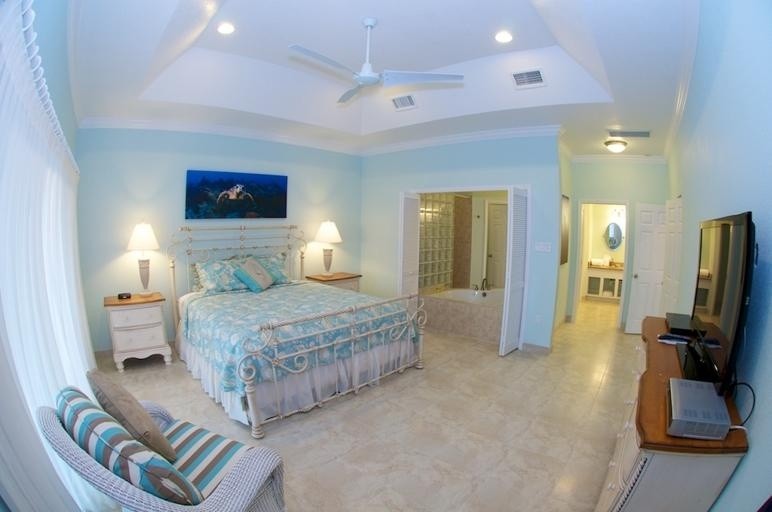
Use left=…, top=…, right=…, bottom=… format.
left=215, top=184, right=255, bottom=206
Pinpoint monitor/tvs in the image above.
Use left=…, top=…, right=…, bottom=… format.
left=676, top=210, right=755, bottom=398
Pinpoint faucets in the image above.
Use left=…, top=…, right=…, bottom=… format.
left=481, top=278, right=487, bottom=290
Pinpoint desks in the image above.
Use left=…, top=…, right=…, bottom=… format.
left=590, top=315, right=749, bottom=511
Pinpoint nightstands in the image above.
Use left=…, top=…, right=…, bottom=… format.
left=104, top=290, right=173, bottom=373
left=304, top=271, right=364, bottom=293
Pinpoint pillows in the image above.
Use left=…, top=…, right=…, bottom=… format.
left=85, top=366, right=178, bottom=465
left=191, top=251, right=295, bottom=297
left=233, top=255, right=279, bottom=294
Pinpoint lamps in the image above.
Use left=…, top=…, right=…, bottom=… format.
left=603, top=140, right=627, bottom=154
left=125, top=221, right=161, bottom=298
left=314, top=220, right=343, bottom=277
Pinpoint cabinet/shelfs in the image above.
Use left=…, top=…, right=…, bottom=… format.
left=584, top=267, right=625, bottom=300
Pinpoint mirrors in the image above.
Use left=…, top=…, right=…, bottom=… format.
left=604, top=222, right=623, bottom=250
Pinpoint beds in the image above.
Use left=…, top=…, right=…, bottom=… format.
left=167, top=224, right=428, bottom=440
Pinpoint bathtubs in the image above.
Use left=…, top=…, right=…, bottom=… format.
left=429, top=289, right=506, bottom=306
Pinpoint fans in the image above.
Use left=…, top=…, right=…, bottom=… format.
left=288, top=18, right=464, bottom=104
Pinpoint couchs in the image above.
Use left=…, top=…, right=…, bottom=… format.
left=36, top=399, right=285, bottom=512
